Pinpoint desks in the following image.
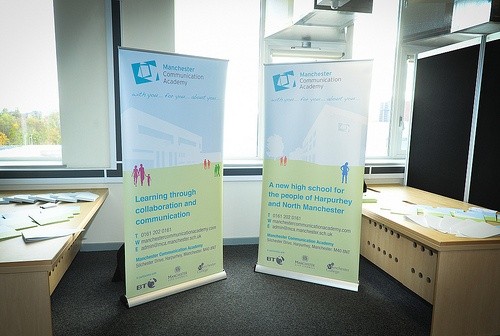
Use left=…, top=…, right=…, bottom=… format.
left=0, top=187, right=110, bottom=336
left=359, top=183, right=500, bottom=335
